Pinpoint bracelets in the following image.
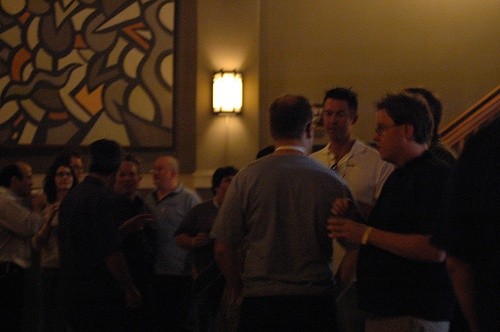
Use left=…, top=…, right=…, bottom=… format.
left=185, top=236, right=193, bottom=248
left=362, top=226, right=373, bottom=245
left=36, top=231, right=46, bottom=239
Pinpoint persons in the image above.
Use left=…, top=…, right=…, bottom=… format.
left=64, top=151, right=84, bottom=184
left=112, top=154, right=157, bottom=332
left=58, top=139, right=154, bottom=332
left=430, top=111, right=500, bottom=332
left=173, top=166, right=240, bottom=332
left=399, top=88, right=460, bottom=168
left=140, top=156, right=202, bottom=332
left=326, top=91, right=454, bottom=332
left=0, top=163, right=48, bottom=332
left=305, top=86, right=399, bottom=332
left=211, top=93, right=366, bottom=332
left=30, top=156, right=83, bottom=332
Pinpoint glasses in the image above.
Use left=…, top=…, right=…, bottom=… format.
left=55, top=171, right=73, bottom=177
left=374, top=123, right=406, bottom=136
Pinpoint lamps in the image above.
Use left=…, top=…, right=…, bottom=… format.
left=212, top=69, right=244, bottom=116
